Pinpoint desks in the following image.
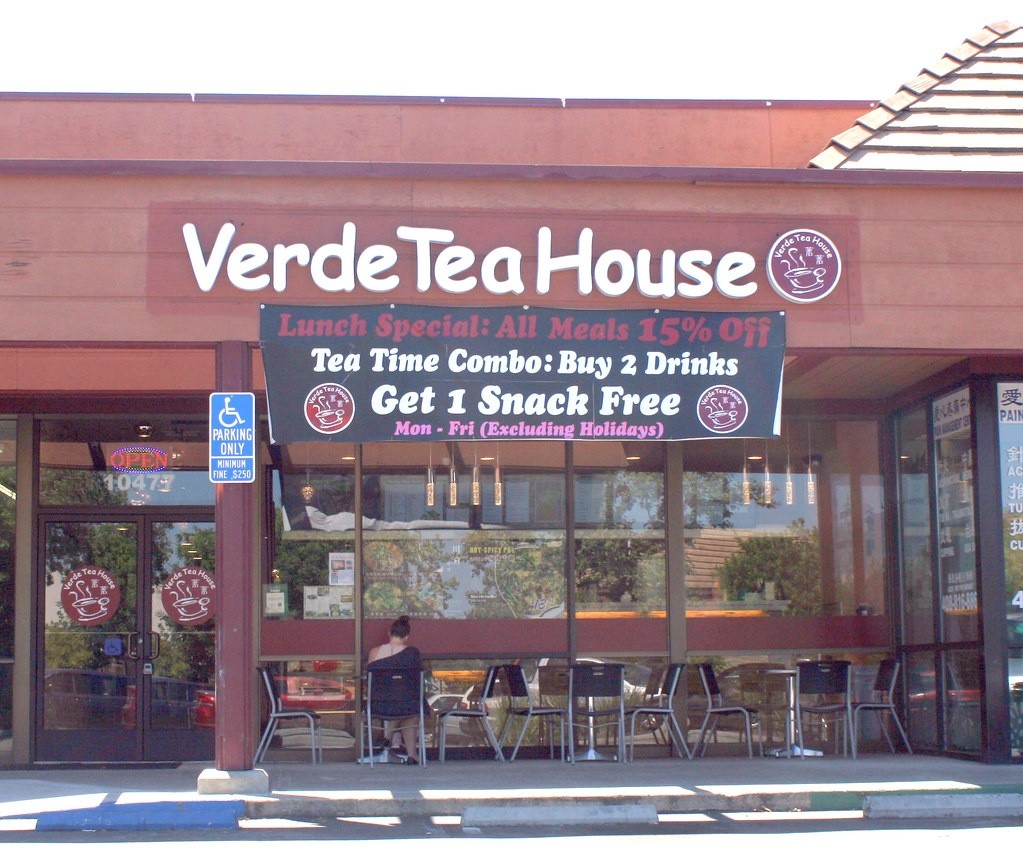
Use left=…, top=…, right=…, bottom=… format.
left=353, top=676, right=435, bottom=764
left=558, top=672, right=633, bottom=762
left=766, top=668, right=869, bottom=757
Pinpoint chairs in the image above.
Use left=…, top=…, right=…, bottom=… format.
left=438, top=666, right=506, bottom=763
left=252, top=667, right=322, bottom=766
left=784, top=659, right=856, bottom=758
left=843, top=659, right=912, bottom=757
left=495, top=664, right=566, bottom=763
left=624, top=664, right=666, bottom=743
left=689, top=662, right=764, bottom=760
left=569, top=663, right=627, bottom=764
left=711, top=697, right=744, bottom=743
left=624, top=663, right=691, bottom=763
left=365, top=665, right=427, bottom=769
left=539, top=666, right=583, bottom=746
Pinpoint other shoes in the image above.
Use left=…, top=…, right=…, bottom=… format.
left=380, top=738, right=392, bottom=748
left=407, top=757, right=418, bottom=765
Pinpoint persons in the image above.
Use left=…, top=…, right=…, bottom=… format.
left=366, top=615, right=429, bottom=765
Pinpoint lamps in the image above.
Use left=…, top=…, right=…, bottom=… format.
left=426, top=442, right=435, bottom=506
left=741, top=438, right=751, bottom=505
left=134, top=421, right=154, bottom=439
left=299, top=468, right=315, bottom=504
left=448, top=441, right=458, bottom=506
left=807, top=421, right=815, bottom=503
left=785, top=421, right=793, bottom=505
left=764, top=439, right=773, bottom=504
left=470, top=442, right=482, bottom=506
left=493, top=441, right=504, bottom=505
